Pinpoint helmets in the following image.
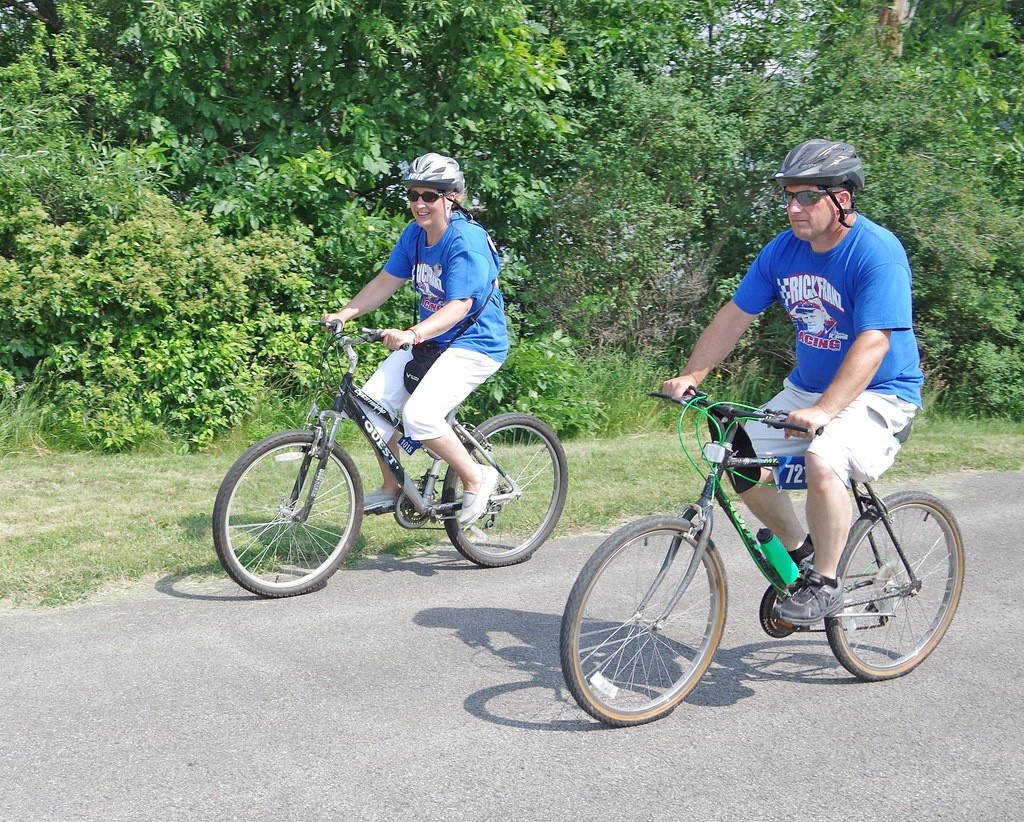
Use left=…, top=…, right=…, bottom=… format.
left=400, top=152, right=465, bottom=196
left=768, top=139, right=864, bottom=192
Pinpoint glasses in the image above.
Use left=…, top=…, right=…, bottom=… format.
left=781, top=190, right=846, bottom=207
left=406, top=188, right=453, bottom=203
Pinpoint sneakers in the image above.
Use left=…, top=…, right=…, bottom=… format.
left=773, top=567, right=844, bottom=627
left=796, top=551, right=815, bottom=573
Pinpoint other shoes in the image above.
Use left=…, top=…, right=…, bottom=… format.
left=362, top=487, right=398, bottom=512
left=459, top=463, right=499, bottom=526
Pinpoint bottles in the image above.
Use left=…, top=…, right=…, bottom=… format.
left=757, top=527, right=798, bottom=585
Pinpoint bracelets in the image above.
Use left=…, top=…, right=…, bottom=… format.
left=407, top=326, right=422, bottom=346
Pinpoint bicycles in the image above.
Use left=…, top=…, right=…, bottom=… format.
left=557, top=388, right=968, bottom=728
left=210, top=315, right=571, bottom=598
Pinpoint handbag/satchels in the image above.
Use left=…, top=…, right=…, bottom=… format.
left=404, top=347, right=441, bottom=394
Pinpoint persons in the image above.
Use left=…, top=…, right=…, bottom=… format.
left=660, top=139, right=923, bottom=629
left=321, top=153, right=509, bottom=532
